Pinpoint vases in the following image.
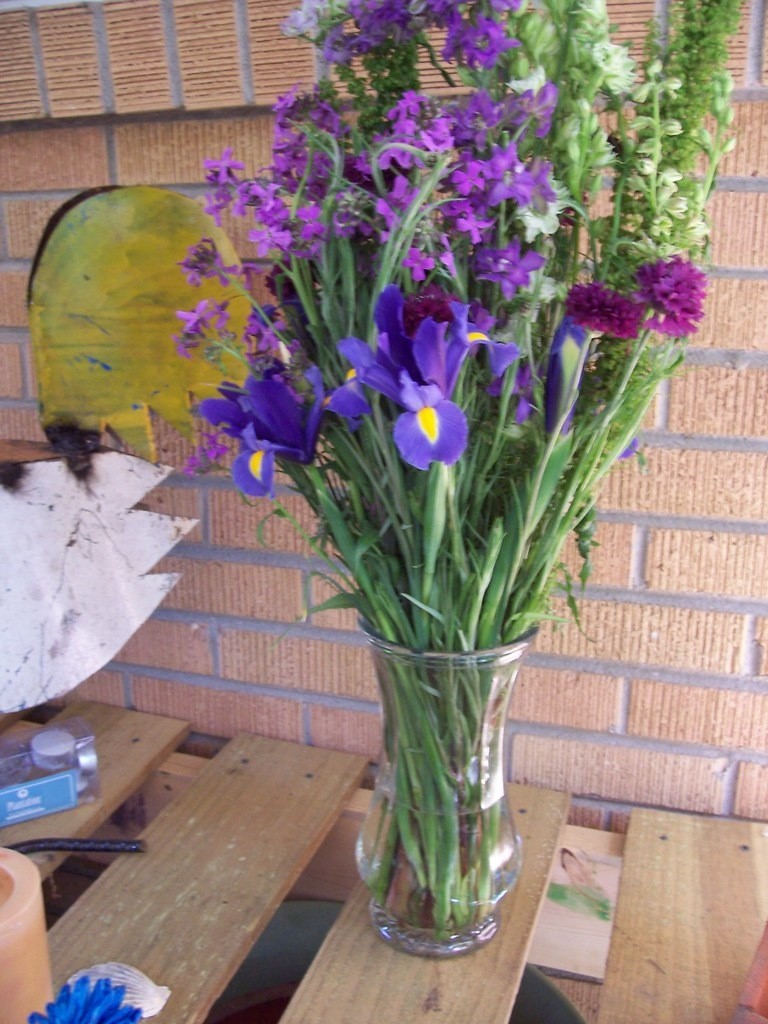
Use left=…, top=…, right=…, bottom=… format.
left=356, top=616, right=539, bottom=960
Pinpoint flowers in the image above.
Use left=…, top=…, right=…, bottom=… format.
left=174, top=0, right=744, bottom=655
